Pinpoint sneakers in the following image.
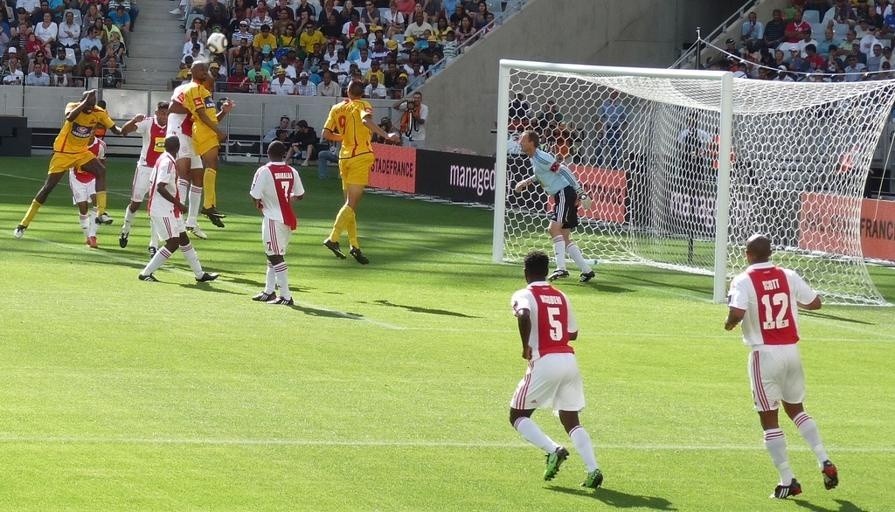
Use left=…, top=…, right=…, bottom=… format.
left=324, top=239, right=347, bottom=259
left=15, top=225, right=26, bottom=238
left=149, top=247, right=158, bottom=258
left=187, top=223, right=207, bottom=239
left=252, top=291, right=276, bottom=301
left=267, top=296, right=293, bottom=304
left=201, top=205, right=226, bottom=218
left=581, top=469, right=602, bottom=488
left=580, top=270, right=595, bottom=282
left=544, top=447, right=569, bottom=482
left=139, top=273, right=158, bottom=282
left=87, top=236, right=90, bottom=245
left=294, top=151, right=301, bottom=157
left=185, top=223, right=193, bottom=231
left=95, top=213, right=112, bottom=223
left=90, top=236, right=96, bottom=247
left=549, top=270, right=570, bottom=281
left=206, top=215, right=224, bottom=228
left=301, top=160, right=309, bottom=167
left=770, top=479, right=802, bottom=499
left=196, top=271, right=218, bottom=282
left=120, top=232, right=129, bottom=248
left=822, top=460, right=838, bottom=490
left=350, top=245, right=369, bottom=264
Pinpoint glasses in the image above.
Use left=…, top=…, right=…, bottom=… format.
left=37, top=55, right=43, bottom=58
left=306, top=26, right=313, bottom=29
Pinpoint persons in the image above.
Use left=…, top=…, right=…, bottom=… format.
left=695, top=1, right=895, bottom=83
left=13, top=89, right=129, bottom=251
left=249, top=139, right=306, bottom=307
left=508, top=246, right=604, bottom=490
left=183, top=70, right=237, bottom=229
left=169, top=2, right=498, bottom=99
left=167, top=60, right=210, bottom=241
left=320, top=78, right=401, bottom=265
left=138, top=135, right=222, bottom=283
left=723, top=231, right=840, bottom=500
left=507, top=89, right=634, bottom=282
left=119, top=101, right=170, bottom=258
left=0, top=0, right=140, bottom=86
left=264, top=90, right=429, bottom=180
left=667, top=108, right=864, bottom=190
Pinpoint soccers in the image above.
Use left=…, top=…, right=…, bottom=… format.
left=207, top=32, right=227, bottom=55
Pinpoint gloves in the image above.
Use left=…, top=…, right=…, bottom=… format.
left=515, top=178, right=533, bottom=192
left=576, top=187, right=592, bottom=209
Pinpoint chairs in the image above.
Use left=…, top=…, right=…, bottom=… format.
left=0, top=0, right=132, bottom=89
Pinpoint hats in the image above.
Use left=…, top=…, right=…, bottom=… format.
left=375, top=26, right=383, bottom=31
left=241, top=21, right=247, bottom=25
left=386, top=40, right=397, bottom=50
left=427, top=36, right=436, bottom=42
left=789, top=48, right=799, bottom=54
left=277, top=69, right=285, bottom=74
left=9, top=47, right=16, bottom=53
left=158, top=100, right=169, bottom=111
left=372, top=38, right=383, bottom=44
left=853, top=40, right=860, bottom=45
left=300, top=72, right=309, bottom=77
left=210, top=62, right=218, bottom=68
left=726, top=39, right=733, bottom=43
left=402, top=37, right=415, bottom=44
left=399, top=74, right=408, bottom=79
left=262, top=45, right=272, bottom=54
left=58, top=46, right=65, bottom=52
left=17, top=7, right=25, bottom=14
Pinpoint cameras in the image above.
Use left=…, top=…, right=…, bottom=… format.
left=405, top=102, right=416, bottom=113
